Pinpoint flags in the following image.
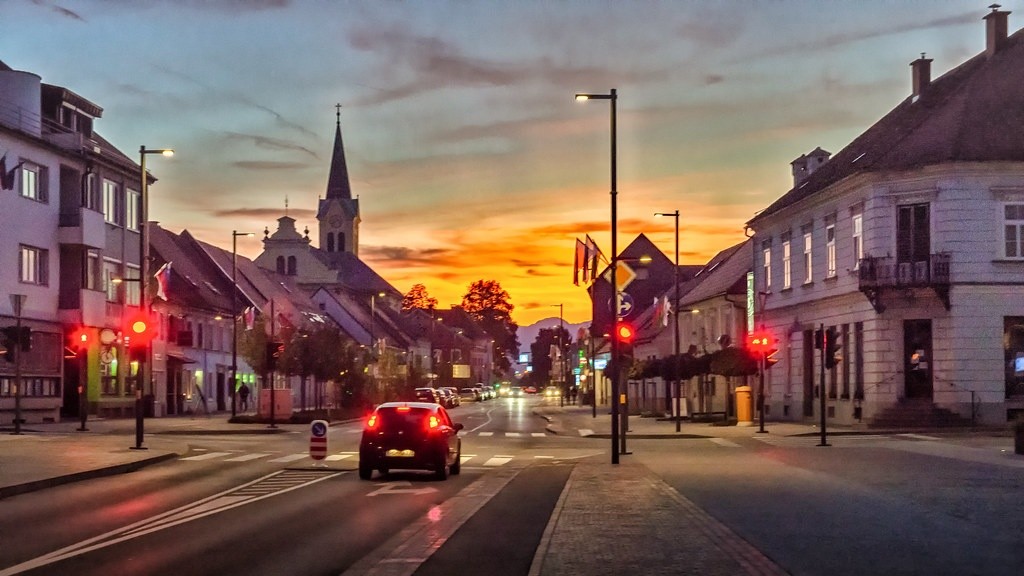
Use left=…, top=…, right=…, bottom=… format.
left=6, top=165, right=20, bottom=190
left=250, top=307, right=255, bottom=329
left=167, top=263, right=172, bottom=288
left=1, top=156, right=7, bottom=190
left=592, top=239, right=602, bottom=279
left=244, top=308, right=251, bottom=330
left=583, top=234, right=602, bottom=285
left=155, top=265, right=169, bottom=301
left=573, top=238, right=597, bottom=286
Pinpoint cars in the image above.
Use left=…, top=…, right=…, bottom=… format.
left=358, top=402, right=464, bottom=481
left=413, top=381, right=562, bottom=409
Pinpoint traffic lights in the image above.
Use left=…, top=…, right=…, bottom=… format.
left=494, top=383, right=500, bottom=388
left=268, top=342, right=284, bottom=370
left=747, top=336, right=771, bottom=349
left=78, top=332, right=90, bottom=345
left=825, top=329, right=843, bottom=370
left=618, top=324, right=634, bottom=340
left=765, top=337, right=781, bottom=371
left=812, top=329, right=820, bottom=349
left=130, top=311, right=155, bottom=341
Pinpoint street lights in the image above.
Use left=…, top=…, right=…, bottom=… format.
left=135, top=143, right=177, bottom=446
left=655, top=209, right=681, bottom=432
left=371, top=291, right=387, bottom=348
left=230, top=230, right=257, bottom=423
left=575, top=87, right=653, bottom=465
